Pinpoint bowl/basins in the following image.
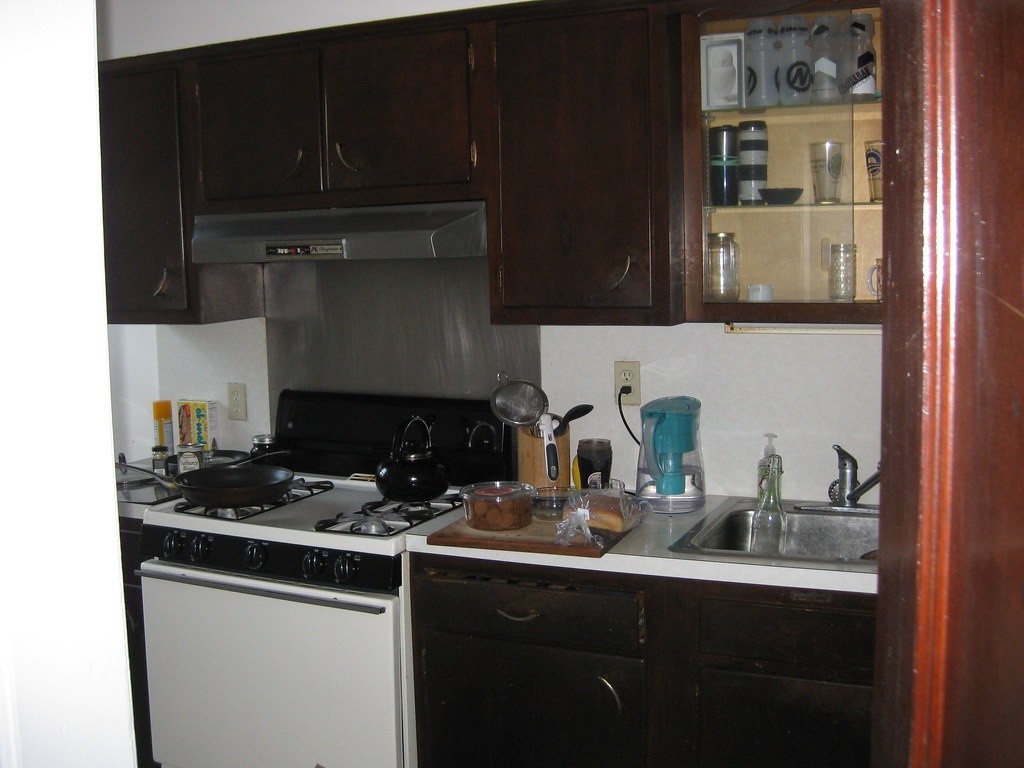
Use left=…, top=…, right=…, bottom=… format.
left=457, top=481, right=536, bottom=531
left=758, top=187, right=803, bottom=205
left=532, top=486, right=579, bottom=522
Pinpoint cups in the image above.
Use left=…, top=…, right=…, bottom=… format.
left=577, top=438, right=613, bottom=490
left=866, top=259, right=884, bottom=303
left=736, top=121, right=768, bottom=207
left=708, top=125, right=740, bottom=206
left=747, top=285, right=775, bottom=304
left=809, top=141, right=846, bottom=205
left=864, top=140, right=883, bottom=204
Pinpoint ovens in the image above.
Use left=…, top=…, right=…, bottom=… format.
left=126, top=526, right=418, bottom=768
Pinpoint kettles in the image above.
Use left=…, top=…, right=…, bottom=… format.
left=635, top=395, right=709, bottom=514
left=376, top=417, right=451, bottom=503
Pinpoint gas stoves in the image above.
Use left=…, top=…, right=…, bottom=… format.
left=143, top=472, right=466, bottom=557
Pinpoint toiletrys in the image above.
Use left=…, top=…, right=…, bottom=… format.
left=757, top=432, right=782, bottom=509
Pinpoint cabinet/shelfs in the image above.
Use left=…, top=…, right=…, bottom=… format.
left=96, top=0, right=890, bottom=326
left=409, top=549, right=879, bottom=768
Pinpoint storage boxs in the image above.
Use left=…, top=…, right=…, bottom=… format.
left=177, top=401, right=220, bottom=450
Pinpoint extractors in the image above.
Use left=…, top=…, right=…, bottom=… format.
left=191, top=200, right=488, bottom=265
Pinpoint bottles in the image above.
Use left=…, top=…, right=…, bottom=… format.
left=151, top=445, right=170, bottom=476
left=708, top=232, right=741, bottom=302
left=250, top=433, right=283, bottom=464
left=746, top=456, right=788, bottom=555
left=827, top=245, right=858, bottom=303
left=176, top=443, right=205, bottom=475
left=745, top=13, right=879, bottom=108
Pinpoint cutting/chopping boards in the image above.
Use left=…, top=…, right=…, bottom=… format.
left=426, top=504, right=640, bottom=559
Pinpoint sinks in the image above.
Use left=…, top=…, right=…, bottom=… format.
left=699, top=508, right=879, bottom=563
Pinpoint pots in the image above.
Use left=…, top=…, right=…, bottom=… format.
left=115, top=462, right=295, bottom=509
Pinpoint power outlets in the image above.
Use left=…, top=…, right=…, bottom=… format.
left=227, top=383, right=247, bottom=421
left=614, top=361, right=641, bottom=405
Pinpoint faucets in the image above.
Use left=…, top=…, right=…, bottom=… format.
left=831, top=444, right=881, bottom=507
